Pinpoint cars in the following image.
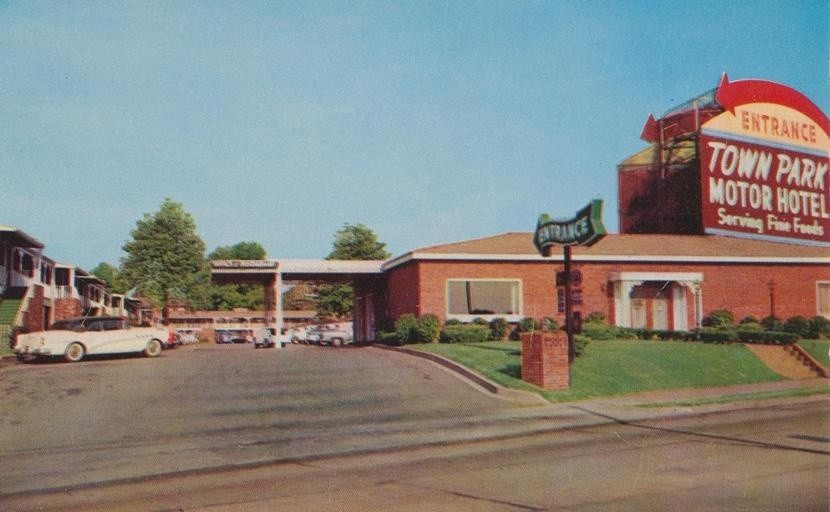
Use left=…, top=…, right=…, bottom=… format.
left=14, top=318, right=175, bottom=361
left=170, top=321, right=353, bottom=347
